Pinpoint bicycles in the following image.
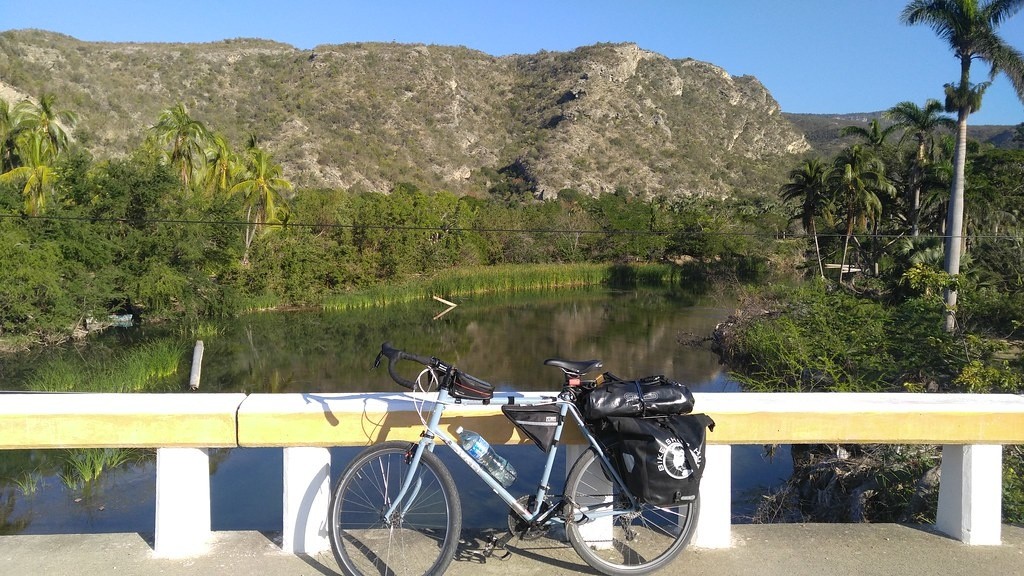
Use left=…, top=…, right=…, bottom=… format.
left=328, top=342, right=716, bottom=576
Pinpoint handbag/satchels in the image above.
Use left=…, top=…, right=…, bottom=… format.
left=580, top=373, right=715, bottom=507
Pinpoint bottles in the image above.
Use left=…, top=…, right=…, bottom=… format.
left=455, top=426, right=518, bottom=488
